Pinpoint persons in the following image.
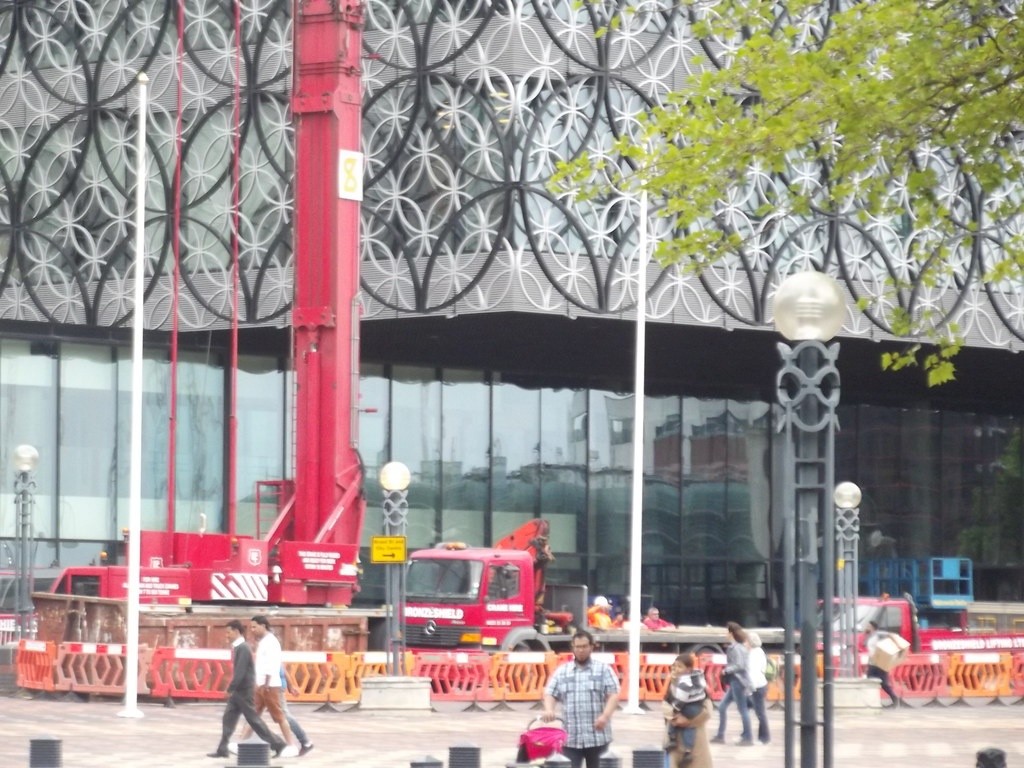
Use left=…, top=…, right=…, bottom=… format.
left=710, top=622, right=753, bottom=745
left=544, top=630, right=620, bottom=768
left=228, top=614, right=298, bottom=757
left=488, top=568, right=499, bottom=599
left=747, top=633, right=771, bottom=745
left=588, top=597, right=649, bottom=631
left=863, top=622, right=904, bottom=708
left=644, top=607, right=674, bottom=630
left=207, top=621, right=287, bottom=758
left=662, top=654, right=713, bottom=768
left=279, top=664, right=314, bottom=756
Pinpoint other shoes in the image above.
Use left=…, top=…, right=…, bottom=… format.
left=282, top=742, right=299, bottom=757
left=207, top=750, right=228, bottom=758
left=226, top=742, right=239, bottom=755
left=682, top=752, right=693, bottom=762
left=300, top=741, right=314, bottom=756
left=738, top=741, right=752, bottom=746
left=755, top=739, right=772, bottom=747
left=711, top=735, right=725, bottom=744
left=272, top=743, right=287, bottom=759
left=664, top=742, right=677, bottom=752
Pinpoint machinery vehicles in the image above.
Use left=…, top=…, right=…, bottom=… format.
left=2, top=2, right=1024, bottom=701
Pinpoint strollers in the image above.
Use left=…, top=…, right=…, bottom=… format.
left=505, top=714, right=568, bottom=767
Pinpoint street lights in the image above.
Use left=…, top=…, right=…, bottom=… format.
left=358, top=461, right=434, bottom=711
left=817, top=481, right=882, bottom=707
left=772, top=272, right=842, bottom=767
left=10, top=444, right=39, bottom=639
